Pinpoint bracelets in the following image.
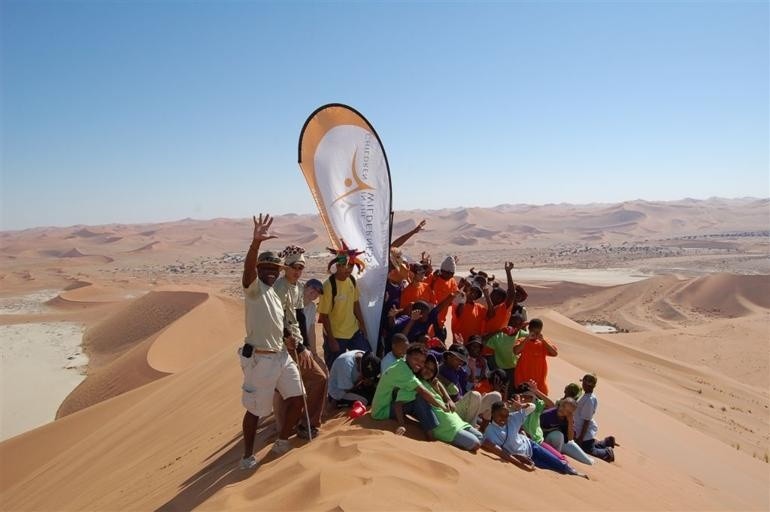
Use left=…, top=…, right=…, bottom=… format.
left=250, top=244, right=259, bottom=250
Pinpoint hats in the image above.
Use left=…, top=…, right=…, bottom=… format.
left=255, top=251, right=288, bottom=269
left=443, top=342, right=469, bottom=365
left=468, top=271, right=490, bottom=300
left=425, top=353, right=439, bottom=381
left=306, top=278, right=323, bottom=295
left=441, top=254, right=457, bottom=273
left=506, top=284, right=528, bottom=304
left=283, top=252, right=306, bottom=268
left=465, top=334, right=485, bottom=347
left=325, top=237, right=367, bottom=275
left=357, top=349, right=381, bottom=381
left=408, top=260, right=429, bottom=272
left=580, top=372, right=597, bottom=387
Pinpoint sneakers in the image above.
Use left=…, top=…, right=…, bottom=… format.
left=271, top=422, right=320, bottom=454
left=240, top=456, right=261, bottom=471
left=606, top=436, right=616, bottom=463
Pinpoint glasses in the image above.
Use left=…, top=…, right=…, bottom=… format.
left=291, top=266, right=304, bottom=270
left=262, top=256, right=281, bottom=263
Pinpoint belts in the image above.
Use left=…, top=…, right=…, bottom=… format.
left=254, top=348, right=278, bottom=354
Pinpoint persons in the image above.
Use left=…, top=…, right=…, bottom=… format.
left=304, top=277, right=330, bottom=378
left=271, top=242, right=327, bottom=439
left=236, top=211, right=306, bottom=471
left=371, top=221, right=619, bottom=480
left=317, top=238, right=372, bottom=371
left=328, top=347, right=381, bottom=408
